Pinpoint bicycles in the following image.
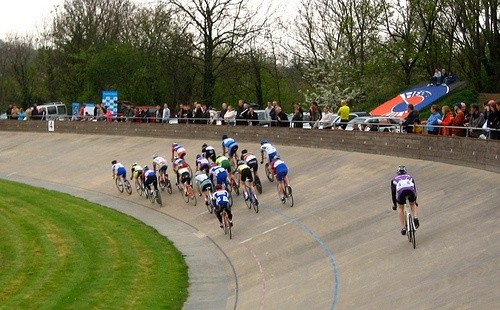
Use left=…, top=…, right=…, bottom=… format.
left=112, top=174, right=132, bottom=195
left=392, top=195, right=419, bottom=249
left=274, top=173, right=294, bottom=207
left=243, top=177, right=259, bottom=213
left=220, top=208, right=232, bottom=239
left=130, top=151, right=275, bottom=214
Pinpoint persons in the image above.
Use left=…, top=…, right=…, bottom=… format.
left=434, top=69, right=448, bottom=88
left=391, top=165, right=419, bottom=235
left=398, top=99, right=500, bottom=140
left=112, top=135, right=291, bottom=228
left=177, top=99, right=259, bottom=126
left=7, top=104, right=38, bottom=120
left=94, top=103, right=170, bottom=123
left=79, top=103, right=86, bottom=119
left=264, top=100, right=378, bottom=130
left=426, top=68, right=441, bottom=88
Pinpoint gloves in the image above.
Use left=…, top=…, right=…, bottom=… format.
left=392, top=205, right=397, bottom=210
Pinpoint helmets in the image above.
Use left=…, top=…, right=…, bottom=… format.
left=153, top=154, right=158, bottom=158
left=260, top=139, right=267, bottom=145
left=132, top=162, right=137, bottom=165
left=241, top=150, right=247, bottom=154
left=216, top=184, right=222, bottom=189
left=221, top=135, right=227, bottom=139
left=172, top=143, right=178, bottom=147
left=272, top=156, right=279, bottom=161
left=174, top=156, right=179, bottom=159
left=196, top=144, right=221, bottom=176
left=396, top=166, right=408, bottom=175
left=238, top=160, right=244, bottom=164
left=112, top=160, right=116, bottom=164
left=143, top=166, right=149, bottom=170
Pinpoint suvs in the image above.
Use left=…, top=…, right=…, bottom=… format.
left=37, top=101, right=68, bottom=120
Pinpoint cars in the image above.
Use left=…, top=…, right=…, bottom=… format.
left=119, top=102, right=408, bottom=133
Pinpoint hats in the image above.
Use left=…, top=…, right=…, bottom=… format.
left=453, top=103, right=462, bottom=109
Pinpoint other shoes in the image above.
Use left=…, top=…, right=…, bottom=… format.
left=414, top=217, right=419, bottom=228
left=245, top=195, right=249, bottom=201
left=401, top=228, right=406, bottom=235
left=282, top=196, right=285, bottom=204
left=230, top=221, right=233, bottom=226
left=220, top=224, right=223, bottom=228
left=288, top=185, right=292, bottom=194
